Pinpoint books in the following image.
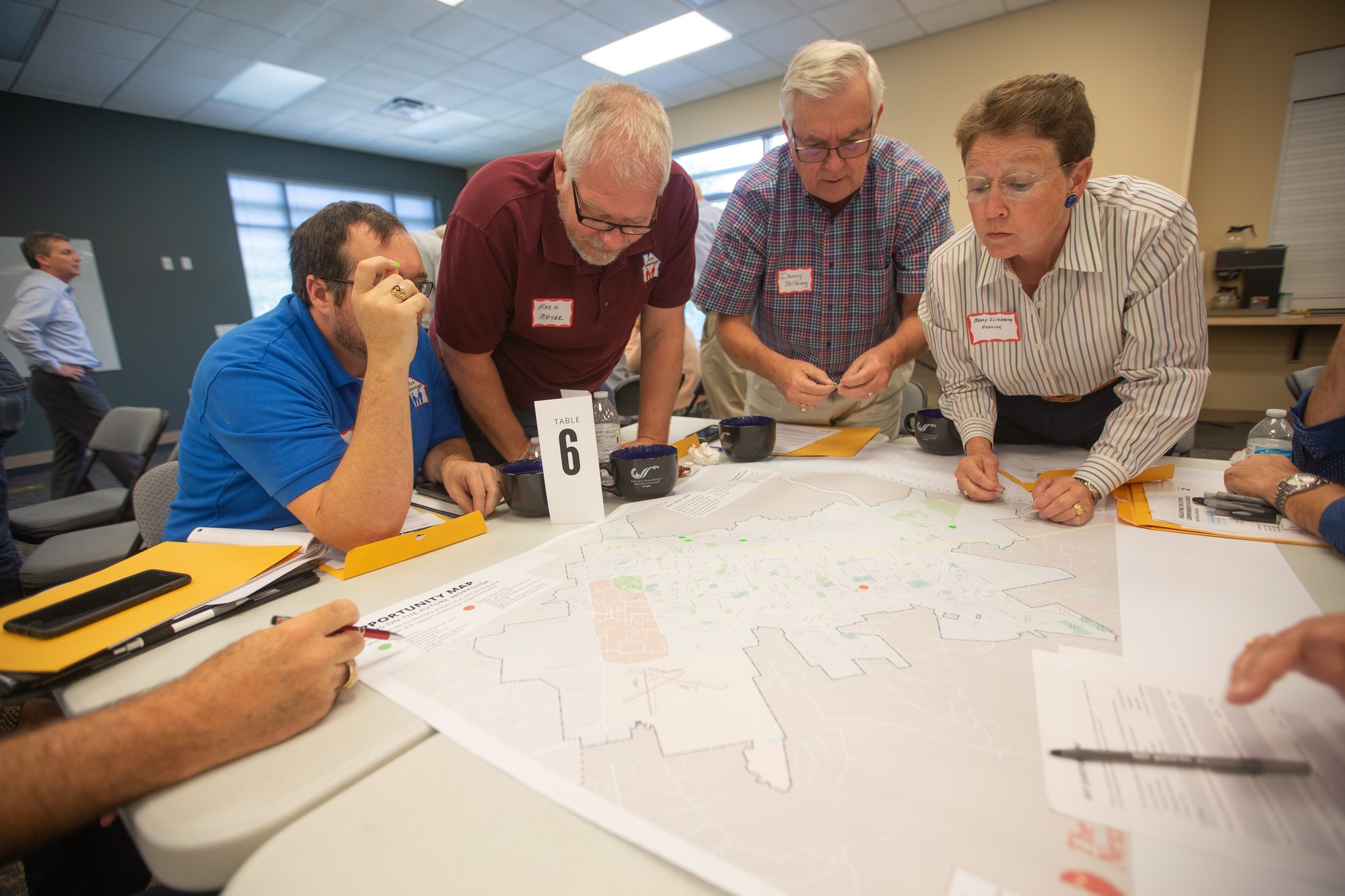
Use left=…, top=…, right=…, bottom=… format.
left=0, top=492, right=486, bottom=674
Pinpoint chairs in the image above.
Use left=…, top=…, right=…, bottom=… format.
left=17, top=506, right=145, bottom=590
left=8, top=407, right=170, bottom=545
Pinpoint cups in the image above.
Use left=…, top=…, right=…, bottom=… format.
left=1250, top=296, right=1269, bottom=310
left=497, top=458, right=549, bottom=518
left=904, top=408, right=964, bottom=456
left=1278, top=292, right=1293, bottom=314
left=718, top=416, right=776, bottom=462
left=599, top=444, right=679, bottom=501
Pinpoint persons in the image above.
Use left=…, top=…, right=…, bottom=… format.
left=4, top=231, right=110, bottom=500
left=160, top=202, right=502, bottom=552
left=0, top=599, right=364, bottom=861
left=691, top=40, right=956, bottom=442
left=427, top=83, right=699, bottom=465
left=1224, top=323, right=1345, bottom=559
left=1226, top=613, right=1345, bottom=703
left=916, top=72, right=1209, bottom=529
left=606, top=182, right=747, bottom=419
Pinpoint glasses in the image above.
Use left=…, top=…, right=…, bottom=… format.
left=792, top=113, right=873, bottom=162
left=572, top=180, right=659, bottom=235
left=958, top=160, right=1075, bottom=202
left=318, top=276, right=433, bottom=299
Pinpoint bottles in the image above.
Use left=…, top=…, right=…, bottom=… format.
left=1245, top=409, right=1294, bottom=464
left=592, top=391, right=622, bottom=486
left=524, top=437, right=541, bottom=459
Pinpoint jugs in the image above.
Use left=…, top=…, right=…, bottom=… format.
left=1225, top=224, right=1258, bottom=249
left=1210, top=285, right=1240, bottom=311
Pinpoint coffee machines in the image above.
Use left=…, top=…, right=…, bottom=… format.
left=1206, top=244, right=1290, bottom=317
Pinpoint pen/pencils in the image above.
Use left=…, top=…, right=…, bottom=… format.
left=1192, top=491, right=1288, bottom=523
left=271, top=615, right=405, bottom=640
left=112, top=597, right=249, bottom=656
left=1051, top=748, right=1310, bottom=775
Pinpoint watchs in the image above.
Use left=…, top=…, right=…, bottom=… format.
left=1075, top=478, right=1100, bottom=506
left=1273, top=473, right=1332, bottom=516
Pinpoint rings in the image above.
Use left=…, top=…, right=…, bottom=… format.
left=1073, top=504, right=1084, bottom=516
left=497, top=481, right=501, bottom=484
left=391, top=285, right=406, bottom=300
left=799, top=403, right=809, bottom=413
left=341, top=659, right=357, bottom=690
left=869, top=392, right=874, bottom=398
left=962, top=490, right=970, bottom=499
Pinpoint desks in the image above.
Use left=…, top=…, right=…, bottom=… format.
left=0, top=413, right=1345, bottom=896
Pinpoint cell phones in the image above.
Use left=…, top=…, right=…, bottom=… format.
left=4, top=570, right=193, bottom=640
left=415, top=482, right=474, bottom=505
left=685, top=425, right=720, bottom=443
